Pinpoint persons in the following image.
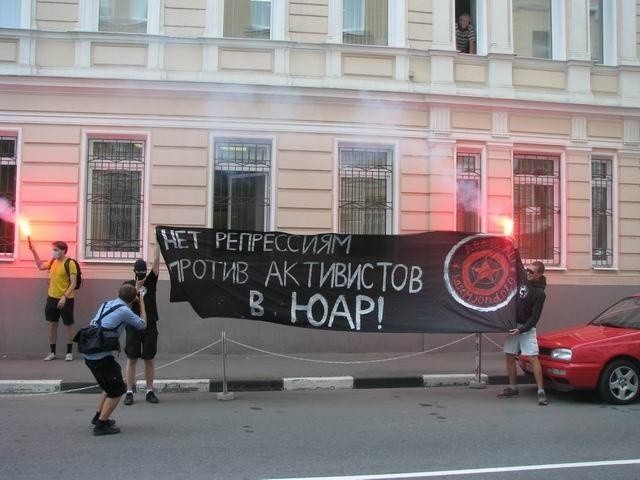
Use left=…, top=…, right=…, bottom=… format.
left=455, top=12, right=476, bottom=54
left=28, top=241, right=78, bottom=361
left=497, top=235, right=547, bottom=405
left=122, top=225, right=161, bottom=405
left=80, top=284, right=148, bottom=435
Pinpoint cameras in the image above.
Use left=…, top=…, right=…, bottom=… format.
left=135, top=286, right=147, bottom=303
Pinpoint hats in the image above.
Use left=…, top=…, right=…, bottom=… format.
left=134, top=259, right=147, bottom=271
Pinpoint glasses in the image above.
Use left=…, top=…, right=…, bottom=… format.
left=526, top=268, right=534, bottom=274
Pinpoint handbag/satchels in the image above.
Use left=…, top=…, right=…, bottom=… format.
left=73, top=301, right=123, bottom=354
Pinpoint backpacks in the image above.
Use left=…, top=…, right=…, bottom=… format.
left=51, top=258, right=81, bottom=289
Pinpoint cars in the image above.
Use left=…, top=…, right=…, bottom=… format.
left=520, top=295, right=639, bottom=404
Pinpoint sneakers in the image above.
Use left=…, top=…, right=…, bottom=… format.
left=65, top=353, right=73, bottom=361
left=124, top=393, right=133, bottom=405
left=497, top=388, right=519, bottom=397
left=92, top=412, right=120, bottom=435
left=44, top=353, right=58, bottom=360
left=145, top=391, right=159, bottom=403
left=538, top=392, right=548, bottom=405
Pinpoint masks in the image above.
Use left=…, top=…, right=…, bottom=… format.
left=52, top=251, right=64, bottom=258
left=135, top=271, right=146, bottom=281
